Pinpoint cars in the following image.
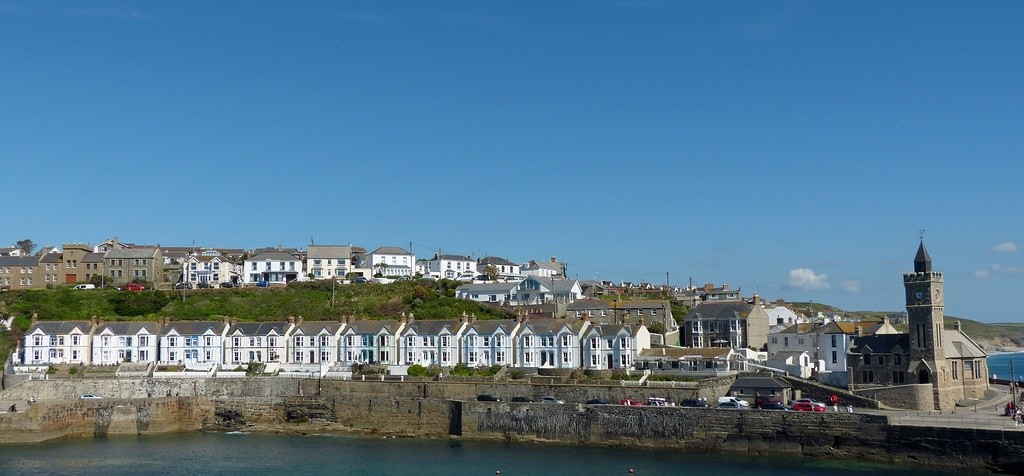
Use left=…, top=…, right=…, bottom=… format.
left=70, top=280, right=272, bottom=291
left=357, top=277, right=367, bottom=282
left=81, top=394, right=104, bottom=400
left=720, top=399, right=749, bottom=409
left=756, top=402, right=789, bottom=411
left=620, top=396, right=710, bottom=408
left=587, top=399, right=613, bottom=405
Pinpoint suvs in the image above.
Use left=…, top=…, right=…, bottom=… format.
left=791, top=398, right=828, bottom=410
left=755, top=395, right=783, bottom=408
left=717, top=397, right=748, bottom=407
left=512, top=397, right=534, bottom=402
left=793, top=401, right=828, bottom=412
left=476, top=395, right=503, bottom=402
left=540, top=396, right=567, bottom=403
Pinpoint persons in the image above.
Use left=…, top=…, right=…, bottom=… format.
left=435, top=372, right=439, bottom=381
left=1005, top=401, right=1011, bottom=412
left=438, top=372, right=442, bottom=381
left=27, top=396, right=36, bottom=406
left=1009, top=382, right=1013, bottom=392
left=7, top=404, right=17, bottom=413
left=992, top=373, right=997, bottom=384
left=166, top=390, right=169, bottom=396
left=169, top=389, right=173, bottom=396
left=1016, top=382, right=1019, bottom=393
